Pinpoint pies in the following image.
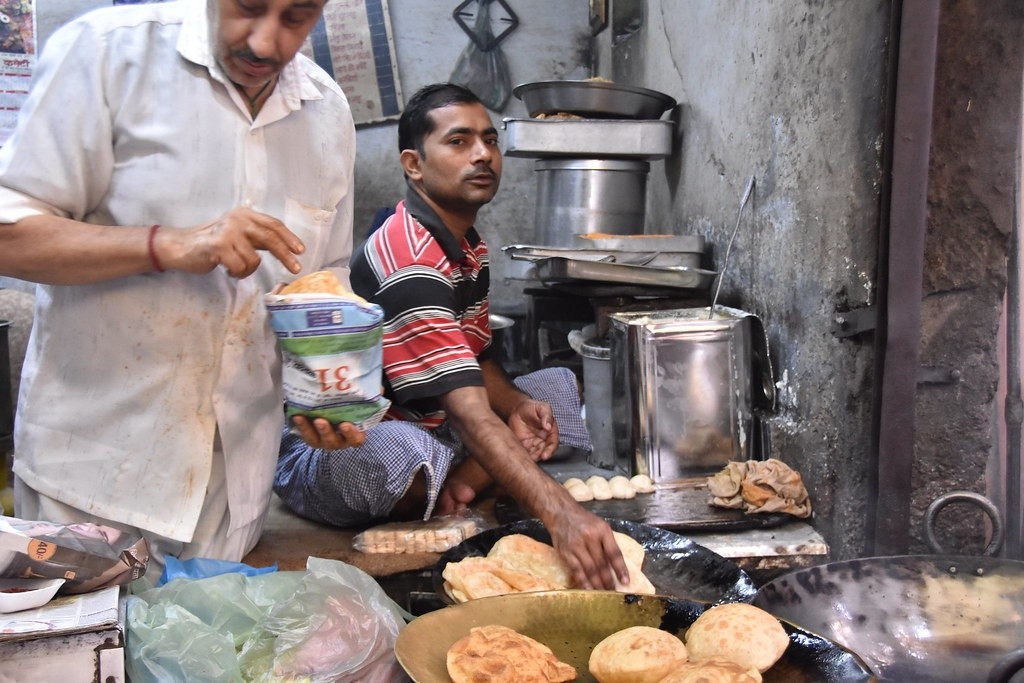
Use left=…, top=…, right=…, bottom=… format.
left=446, top=601, right=790, bottom=683
left=443, top=529, right=655, bottom=603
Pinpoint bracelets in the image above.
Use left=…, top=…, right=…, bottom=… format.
left=148, top=225, right=167, bottom=272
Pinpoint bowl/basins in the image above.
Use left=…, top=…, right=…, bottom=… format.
left=512, top=79, right=677, bottom=120
left=478, top=314, right=515, bottom=361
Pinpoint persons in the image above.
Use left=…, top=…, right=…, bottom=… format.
left=0, top=0, right=365, bottom=562
left=274, top=83, right=631, bottom=590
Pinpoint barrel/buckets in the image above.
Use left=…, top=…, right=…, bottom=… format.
left=533, top=160, right=650, bottom=282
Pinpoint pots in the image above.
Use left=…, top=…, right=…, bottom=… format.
left=747, top=490, right=1024, bottom=683
left=430, top=514, right=757, bottom=606
left=393, top=589, right=878, bottom=683
